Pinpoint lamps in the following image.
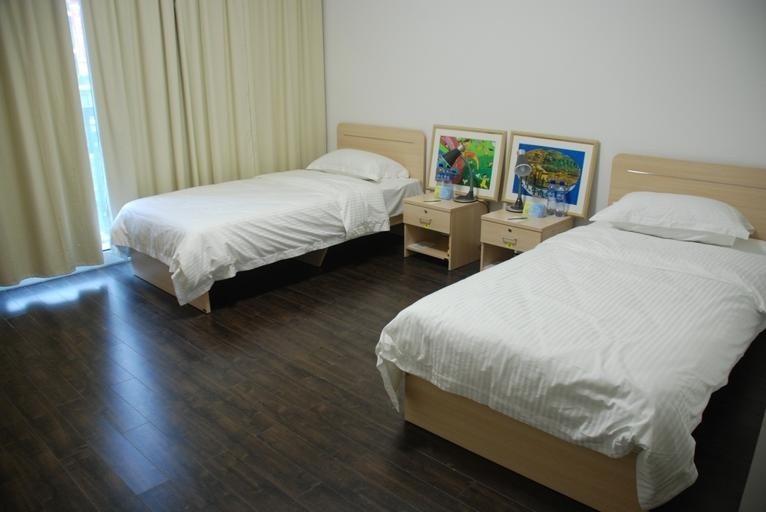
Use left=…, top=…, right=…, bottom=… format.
left=439, top=145, right=478, bottom=204
left=511, top=153, right=530, bottom=213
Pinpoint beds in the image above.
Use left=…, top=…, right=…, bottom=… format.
left=108, top=120, right=435, bottom=313
left=373, top=149, right=765, bottom=509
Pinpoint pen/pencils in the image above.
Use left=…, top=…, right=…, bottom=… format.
left=507, top=217, right=527, bottom=220
left=423, top=199, right=441, bottom=202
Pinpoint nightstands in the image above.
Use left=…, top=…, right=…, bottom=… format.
left=478, top=204, right=575, bottom=274
left=400, top=187, right=488, bottom=271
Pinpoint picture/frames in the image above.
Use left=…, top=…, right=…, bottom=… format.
left=424, top=124, right=508, bottom=205
left=501, top=128, right=601, bottom=221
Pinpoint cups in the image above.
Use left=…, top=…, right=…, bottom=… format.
left=529, top=203, right=547, bottom=217
left=434, top=183, right=440, bottom=196
left=441, top=185, right=451, bottom=199
left=522, top=200, right=532, bottom=214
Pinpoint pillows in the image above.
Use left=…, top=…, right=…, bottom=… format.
left=590, top=190, right=757, bottom=247
left=301, top=146, right=413, bottom=184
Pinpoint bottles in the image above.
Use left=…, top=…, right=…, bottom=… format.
left=435, top=163, right=445, bottom=198
left=545, top=180, right=557, bottom=216
left=556, top=180, right=566, bottom=217
left=441, top=165, right=453, bottom=200
